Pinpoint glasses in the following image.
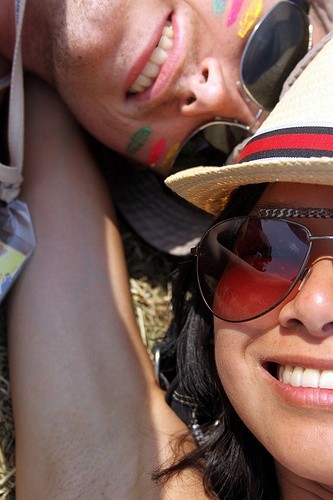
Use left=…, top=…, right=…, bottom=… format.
left=191, top=216, right=333, bottom=322
left=166, top=0, right=312, bottom=178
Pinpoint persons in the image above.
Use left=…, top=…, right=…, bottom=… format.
left=0, top=0, right=327, bottom=205
left=10, top=41, right=333, bottom=500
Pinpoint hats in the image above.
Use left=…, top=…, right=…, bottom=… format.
left=164, top=39, right=333, bottom=216
left=93, top=0, right=333, bottom=256
